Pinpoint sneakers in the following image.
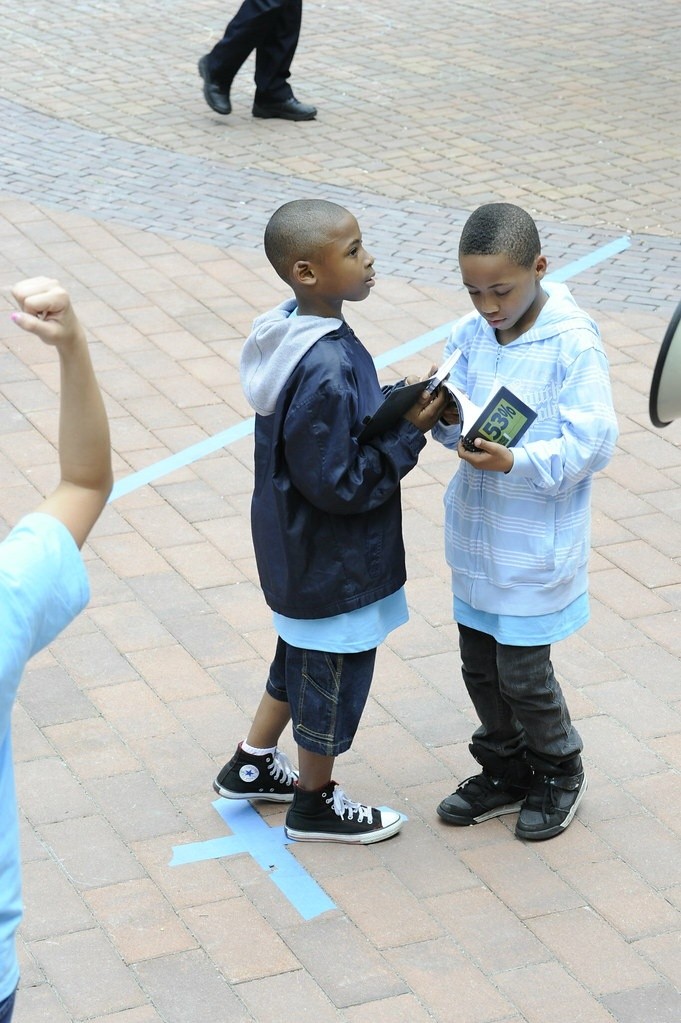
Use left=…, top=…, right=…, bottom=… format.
left=212, top=742, right=301, bottom=802
left=285, top=781, right=403, bottom=846
left=437, top=770, right=529, bottom=825
left=515, top=754, right=586, bottom=838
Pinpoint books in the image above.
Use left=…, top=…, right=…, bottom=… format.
left=357, top=347, right=462, bottom=442
left=443, top=382, right=538, bottom=452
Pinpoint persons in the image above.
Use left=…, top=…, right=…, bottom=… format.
left=0, top=276, right=113, bottom=1023
left=197, top=0, right=318, bottom=120
left=213, top=199, right=449, bottom=845
left=437, top=203, right=618, bottom=841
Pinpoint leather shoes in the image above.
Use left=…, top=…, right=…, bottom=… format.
left=252, top=93, right=315, bottom=120
left=198, top=54, right=233, bottom=114
